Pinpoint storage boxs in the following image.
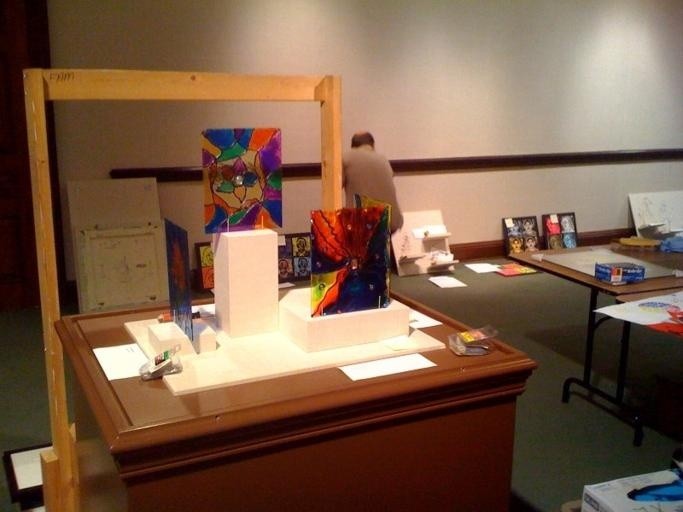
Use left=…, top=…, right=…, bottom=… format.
left=594, top=261, right=645, bottom=286
left=579, top=470, right=683, bottom=512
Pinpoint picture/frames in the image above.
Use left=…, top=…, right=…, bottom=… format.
left=502, top=216, right=540, bottom=258
left=541, top=211, right=578, bottom=250
left=277, top=232, right=313, bottom=284
left=70, top=220, right=172, bottom=316
left=194, top=242, right=217, bottom=292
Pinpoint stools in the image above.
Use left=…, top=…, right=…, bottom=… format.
left=4, top=442, right=53, bottom=511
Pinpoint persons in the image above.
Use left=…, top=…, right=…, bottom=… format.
left=342, top=131, right=403, bottom=234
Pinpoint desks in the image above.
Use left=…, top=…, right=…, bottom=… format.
left=507, top=243, right=683, bottom=445
left=615, top=286, right=682, bottom=446
left=54, top=277, right=538, bottom=511
